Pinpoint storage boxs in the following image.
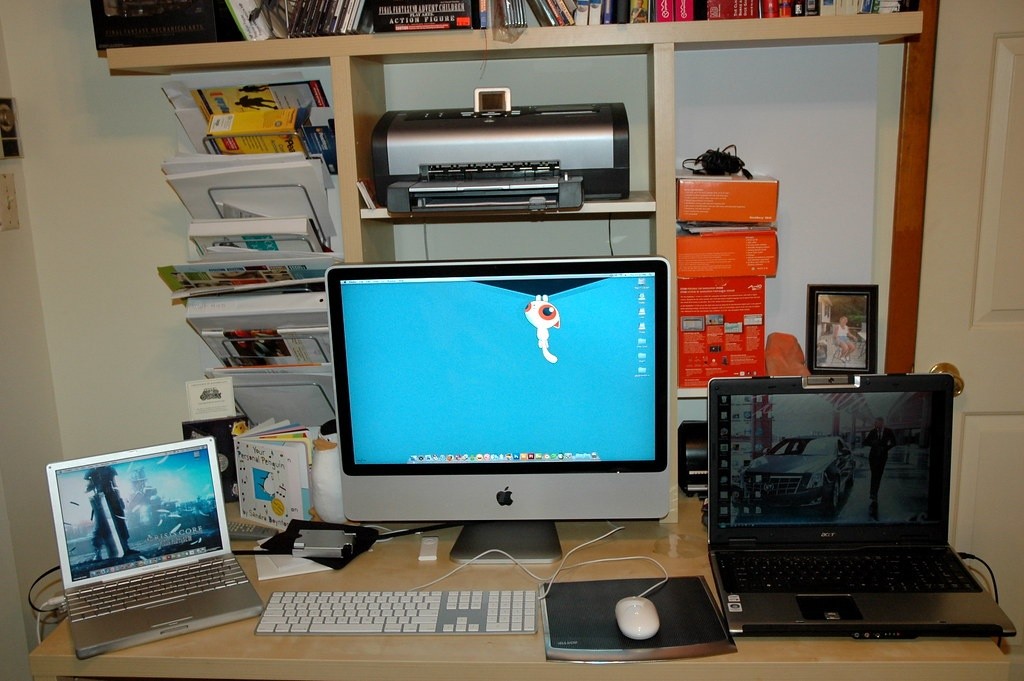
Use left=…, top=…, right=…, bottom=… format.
left=677, top=229, right=779, bottom=279
left=677, top=276, right=768, bottom=388
left=674, top=170, right=780, bottom=224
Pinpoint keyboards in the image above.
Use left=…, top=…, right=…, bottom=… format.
left=255, top=591, right=538, bottom=636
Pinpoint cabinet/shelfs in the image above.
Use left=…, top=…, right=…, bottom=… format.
left=108, top=0, right=939, bottom=404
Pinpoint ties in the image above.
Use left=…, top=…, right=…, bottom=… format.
left=877, top=430, right=882, bottom=438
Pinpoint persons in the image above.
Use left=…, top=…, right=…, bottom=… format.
left=833, top=317, right=858, bottom=362
left=863, top=418, right=896, bottom=499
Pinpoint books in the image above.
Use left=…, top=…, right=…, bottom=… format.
left=157, top=74, right=337, bottom=432
left=233, top=418, right=315, bottom=530
left=226, top=0, right=924, bottom=45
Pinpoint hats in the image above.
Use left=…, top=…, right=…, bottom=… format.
left=765, top=333, right=811, bottom=377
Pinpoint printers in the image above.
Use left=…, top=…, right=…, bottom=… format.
left=370, top=87, right=631, bottom=213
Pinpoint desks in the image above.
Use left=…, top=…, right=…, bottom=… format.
left=25, top=495, right=1024, bottom=681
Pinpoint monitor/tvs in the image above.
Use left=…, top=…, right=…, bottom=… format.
left=325, top=254, right=671, bottom=565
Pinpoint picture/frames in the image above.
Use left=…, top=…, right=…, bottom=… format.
left=807, top=283, right=879, bottom=374
left=809, top=284, right=878, bottom=373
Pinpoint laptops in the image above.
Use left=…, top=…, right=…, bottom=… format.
left=45, top=436, right=264, bottom=660
left=708, top=372, right=1018, bottom=639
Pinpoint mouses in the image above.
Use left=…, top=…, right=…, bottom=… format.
left=615, top=595, right=659, bottom=640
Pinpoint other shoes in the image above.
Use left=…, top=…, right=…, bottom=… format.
left=840, top=357, right=846, bottom=362
left=869, top=501, right=878, bottom=521
left=846, top=356, right=849, bottom=361
